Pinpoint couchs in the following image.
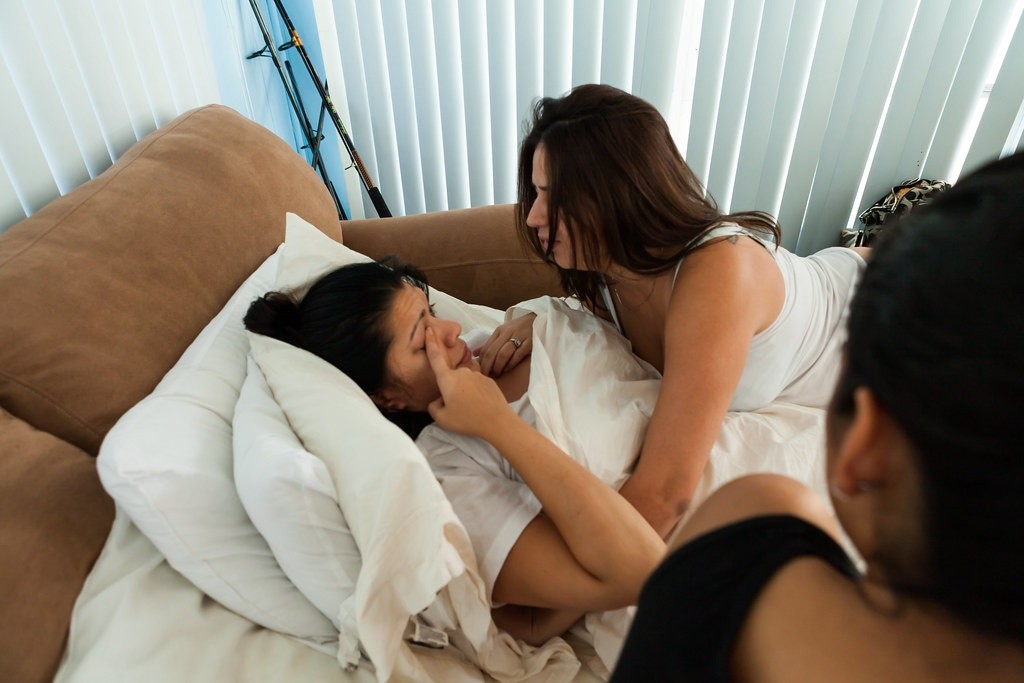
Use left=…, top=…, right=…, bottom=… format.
left=0, top=106, right=559, bottom=683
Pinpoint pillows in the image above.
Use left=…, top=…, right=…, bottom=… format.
left=98, top=208, right=506, bottom=634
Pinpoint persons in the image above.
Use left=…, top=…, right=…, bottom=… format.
left=492, top=84, right=871, bottom=645
left=604, top=148, right=1024, bottom=683
left=244, top=256, right=667, bottom=610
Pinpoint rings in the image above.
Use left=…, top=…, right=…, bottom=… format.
left=510, top=338, right=520, bottom=346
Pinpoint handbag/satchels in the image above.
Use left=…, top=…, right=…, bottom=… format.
left=857, top=176, right=952, bottom=245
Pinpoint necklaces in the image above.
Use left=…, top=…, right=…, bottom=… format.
left=612, top=272, right=658, bottom=311
